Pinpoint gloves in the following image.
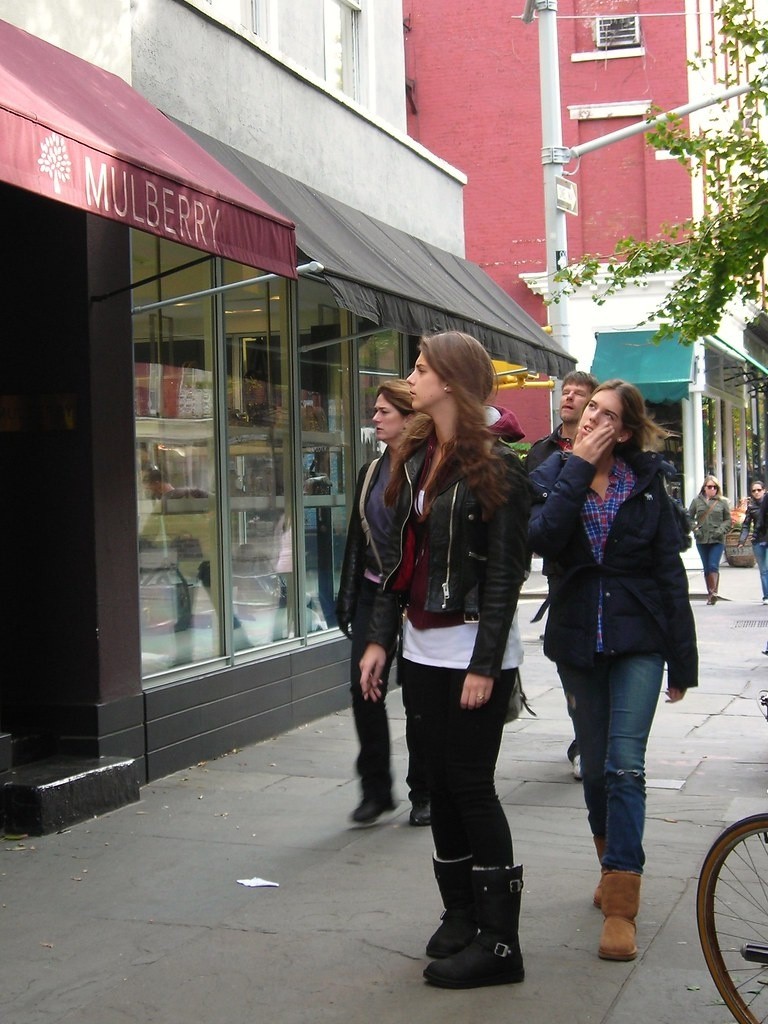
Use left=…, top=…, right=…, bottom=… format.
left=337, top=610, right=354, bottom=643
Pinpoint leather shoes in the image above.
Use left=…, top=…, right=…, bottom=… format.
left=349, top=792, right=399, bottom=823
left=407, top=798, right=434, bottom=827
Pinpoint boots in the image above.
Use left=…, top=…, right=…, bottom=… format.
left=590, top=835, right=606, bottom=908
left=705, top=572, right=719, bottom=606
left=425, top=859, right=528, bottom=987
left=425, top=851, right=472, bottom=958
left=597, top=869, right=643, bottom=962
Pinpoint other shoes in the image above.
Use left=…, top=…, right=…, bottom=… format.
left=762, top=599, right=768, bottom=605
left=572, top=755, right=582, bottom=780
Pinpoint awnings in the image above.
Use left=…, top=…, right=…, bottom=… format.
left=0, top=19, right=299, bottom=282
left=159, top=113, right=578, bottom=381
left=710, top=332, right=768, bottom=377
left=590, top=330, right=693, bottom=404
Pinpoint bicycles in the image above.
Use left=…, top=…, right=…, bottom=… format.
left=696, top=812, right=768, bottom=1024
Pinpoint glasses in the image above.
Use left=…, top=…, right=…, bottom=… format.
left=751, top=488, right=764, bottom=493
left=705, top=484, right=718, bottom=491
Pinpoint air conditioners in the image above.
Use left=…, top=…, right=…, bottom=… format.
left=596, top=15, right=640, bottom=48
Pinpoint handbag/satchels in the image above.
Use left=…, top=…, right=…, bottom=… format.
left=503, top=670, right=536, bottom=724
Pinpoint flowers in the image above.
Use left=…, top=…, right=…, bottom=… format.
left=726, top=496, right=754, bottom=533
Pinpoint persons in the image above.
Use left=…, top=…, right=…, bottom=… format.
left=139, top=467, right=255, bottom=673
left=737, top=480, right=768, bottom=605
left=522, top=372, right=698, bottom=962
left=335, top=332, right=532, bottom=989
left=688, top=475, right=732, bottom=605
left=273, top=491, right=322, bottom=640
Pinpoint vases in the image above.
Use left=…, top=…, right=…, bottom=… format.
left=725, top=532, right=756, bottom=568
